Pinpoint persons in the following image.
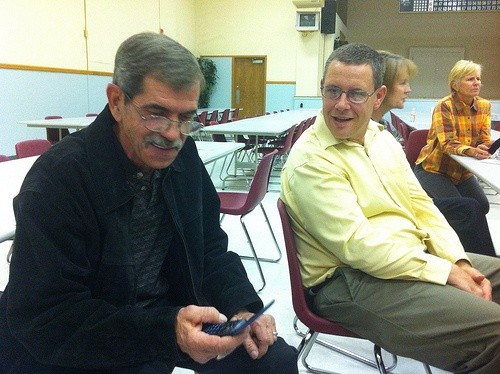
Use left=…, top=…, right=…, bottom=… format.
left=370, top=50, right=498, bottom=257
left=0, top=32, right=299, bottom=374
left=280, top=44, right=500, bottom=374
left=412, top=60, right=492, bottom=216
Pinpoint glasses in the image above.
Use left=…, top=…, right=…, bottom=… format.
left=320, top=80, right=377, bottom=104
left=124, top=93, right=204, bottom=137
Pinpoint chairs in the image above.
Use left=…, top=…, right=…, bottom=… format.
left=0, top=97, right=500, bottom=374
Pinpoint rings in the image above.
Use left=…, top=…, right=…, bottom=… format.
left=476, top=153, right=478, bottom=157
left=273, top=332, right=278, bottom=336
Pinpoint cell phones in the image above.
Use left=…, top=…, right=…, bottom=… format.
left=201, top=299, right=275, bottom=336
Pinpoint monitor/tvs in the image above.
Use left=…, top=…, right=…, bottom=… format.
left=295, top=12, right=319, bottom=31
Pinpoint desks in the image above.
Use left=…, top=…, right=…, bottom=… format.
left=0, top=140, right=244, bottom=292
left=451, top=129, right=500, bottom=196
left=16, top=116, right=97, bottom=140
left=201, top=106, right=321, bottom=180
left=389, top=108, right=432, bottom=130
